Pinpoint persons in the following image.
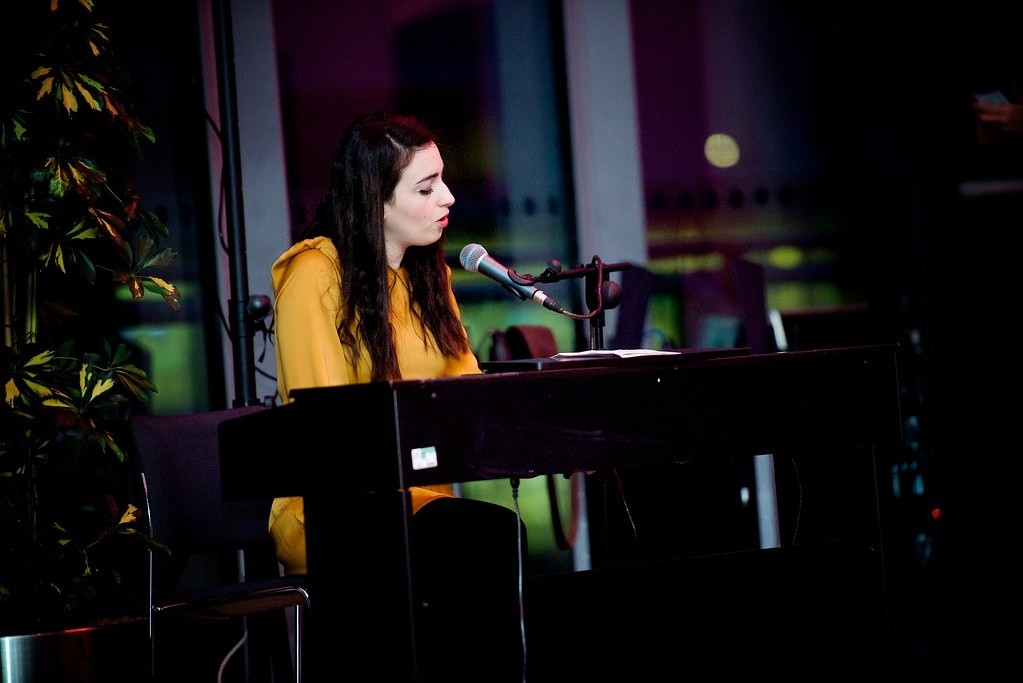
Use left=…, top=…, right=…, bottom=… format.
left=266, top=112, right=528, bottom=683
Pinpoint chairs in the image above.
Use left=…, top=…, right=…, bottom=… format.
left=134, top=262, right=766, bottom=682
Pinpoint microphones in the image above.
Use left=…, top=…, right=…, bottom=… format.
left=460, top=244, right=563, bottom=313
